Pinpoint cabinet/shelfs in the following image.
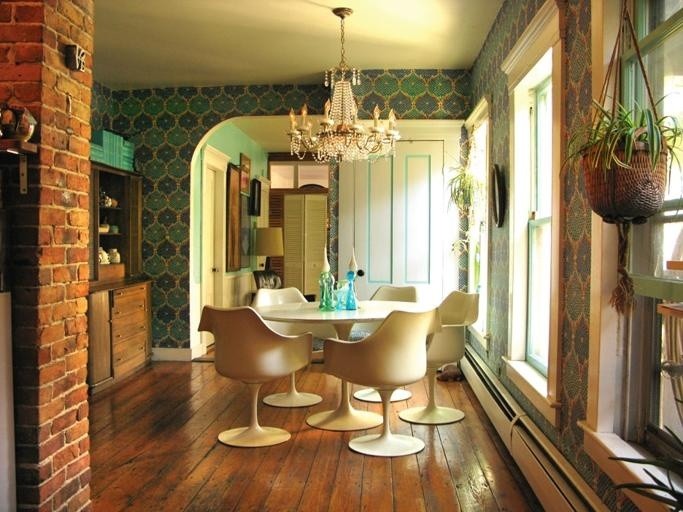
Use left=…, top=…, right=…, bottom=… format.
left=82, top=157, right=155, bottom=407
left=268, top=187, right=328, bottom=296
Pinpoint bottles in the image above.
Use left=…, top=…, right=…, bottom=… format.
left=106, top=247, right=120, bottom=263
left=98, top=246, right=108, bottom=264
left=316, top=270, right=357, bottom=311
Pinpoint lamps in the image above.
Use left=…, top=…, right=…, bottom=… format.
left=251, top=226, right=287, bottom=272
left=281, top=5, right=405, bottom=170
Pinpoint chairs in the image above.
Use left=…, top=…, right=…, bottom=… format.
left=334, top=285, right=419, bottom=404
left=319, top=304, right=438, bottom=459
left=249, top=286, right=323, bottom=411
left=394, top=287, right=480, bottom=426
left=196, top=303, right=315, bottom=450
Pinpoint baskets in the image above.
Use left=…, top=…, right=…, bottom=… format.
left=579, top=132, right=669, bottom=226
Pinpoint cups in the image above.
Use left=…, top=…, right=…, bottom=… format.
left=110, top=226, right=118, bottom=233
left=111, top=200, right=117, bottom=207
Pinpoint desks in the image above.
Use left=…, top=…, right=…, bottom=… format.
left=256, top=298, right=437, bottom=434
left=248, top=268, right=317, bottom=307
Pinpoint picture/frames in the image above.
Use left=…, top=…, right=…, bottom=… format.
left=237, top=193, right=253, bottom=269
left=237, top=152, right=252, bottom=198
left=487, top=160, right=508, bottom=230
left=246, top=178, right=261, bottom=217
left=224, top=161, right=243, bottom=273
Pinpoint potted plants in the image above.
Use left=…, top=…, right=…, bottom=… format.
left=554, top=0, right=683, bottom=227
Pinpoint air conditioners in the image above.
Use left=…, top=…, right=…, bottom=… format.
left=458, top=342, right=528, bottom=457
left=507, top=410, right=616, bottom=512
